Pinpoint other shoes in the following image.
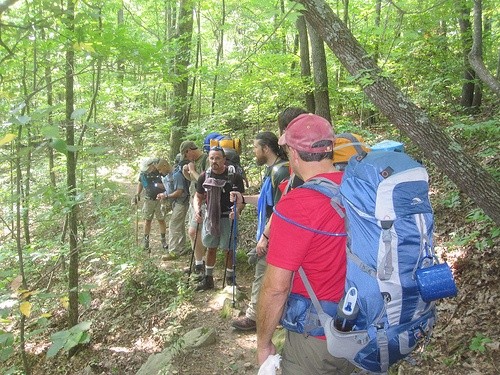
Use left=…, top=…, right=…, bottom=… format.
left=161, top=238, right=168, bottom=248
left=142, top=240, right=150, bottom=251
left=231, top=315, right=257, bottom=331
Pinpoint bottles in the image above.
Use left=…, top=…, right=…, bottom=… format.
left=333, top=287, right=359, bottom=331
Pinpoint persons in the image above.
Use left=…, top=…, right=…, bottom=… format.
left=233, top=132, right=291, bottom=331
left=152, top=157, right=189, bottom=261
left=180, top=141, right=209, bottom=277
left=135, top=157, right=169, bottom=250
left=273, top=106, right=309, bottom=205
left=256, top=113, right=365, bottom=375
left=193, top=146, right=245, bottom=291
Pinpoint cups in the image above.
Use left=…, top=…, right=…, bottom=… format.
left=416, top=255, right=457, bottom=302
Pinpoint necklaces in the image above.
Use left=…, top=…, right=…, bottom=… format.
left=273, top=156, right=279, bottom=165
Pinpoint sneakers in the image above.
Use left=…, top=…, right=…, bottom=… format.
left=195, top=275, right=214, bottom=290
left=226, top=275, right=241, bottom=290
left=194, top=261, right=206, bottom=276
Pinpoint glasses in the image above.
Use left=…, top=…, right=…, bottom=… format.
left=211, top=146, right=222, bottom=151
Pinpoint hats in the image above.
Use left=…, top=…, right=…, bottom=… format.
left=179, top=141, right=198, bottom=161
left=278, top=113, right=337, bottom=154
left=140, top=157, right=154, bottom=172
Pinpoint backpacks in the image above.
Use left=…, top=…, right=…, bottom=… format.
left=332, top=134, right=370, bottom=171
left=222, top=147, right=250, bottom=187
left=174, top=153, right=193, bottom=197
left=202, top=131, right=241, bottom=153
left=294, top=151, right=457, bottom=374
